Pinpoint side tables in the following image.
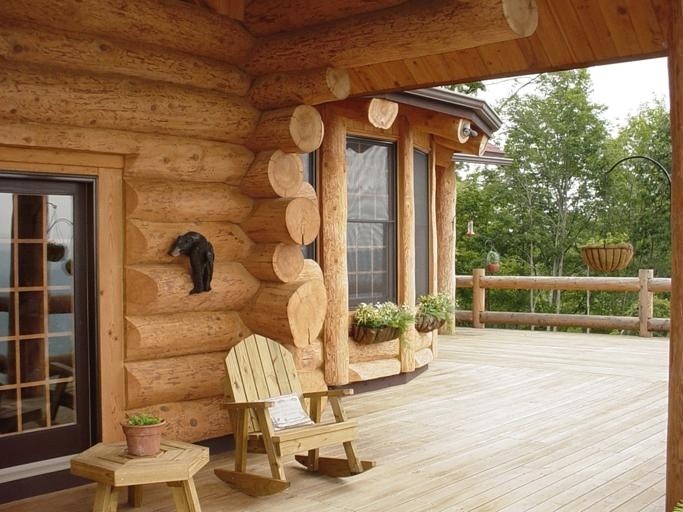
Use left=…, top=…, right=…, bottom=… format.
left=0, top=401, right=42, bottom=433
left=70, top=441, right=210, bottom=512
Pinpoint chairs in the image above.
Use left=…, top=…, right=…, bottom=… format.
left=51, top=363, right=73, bottom=420
left=214, top=334, right=376, bottom=497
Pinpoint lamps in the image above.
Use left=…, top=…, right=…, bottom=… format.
left=461, top=123, right=478, bottom=139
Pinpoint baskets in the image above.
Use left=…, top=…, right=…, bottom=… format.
left=580, top=244, right=634, bottom=274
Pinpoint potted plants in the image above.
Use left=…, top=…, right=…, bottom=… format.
left=576, top=232, right=634, bottom=273
left=415, top=294, right=460, bottom=335
left=47, top=242, right=65, bottom=262
left=487, top=251, right=501, bottom=273
left=352, top=301, right=419, bottom=345
left=120, top=414, right=166, bottom=457
